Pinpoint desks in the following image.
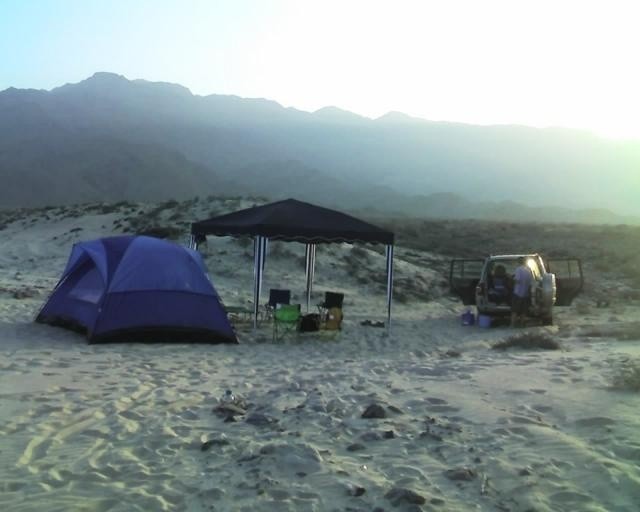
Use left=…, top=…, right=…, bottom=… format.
left=221, top=297, right=263, bottom=324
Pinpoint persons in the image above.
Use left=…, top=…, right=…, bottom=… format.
left=507, top=257, right=535, bottom=330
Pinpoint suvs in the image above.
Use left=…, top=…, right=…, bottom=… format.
left=448, top=252, right=584, bottom=326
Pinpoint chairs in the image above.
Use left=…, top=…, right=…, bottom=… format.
left=264, top=289, right=344, bottom=343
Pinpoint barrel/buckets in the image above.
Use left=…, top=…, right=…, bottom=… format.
left=478, top=314, right=493, bottom=329
left=461, top=310, right=475, bottom=326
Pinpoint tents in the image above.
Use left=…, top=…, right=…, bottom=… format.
left=34, top=233, right=239, bottom=344
